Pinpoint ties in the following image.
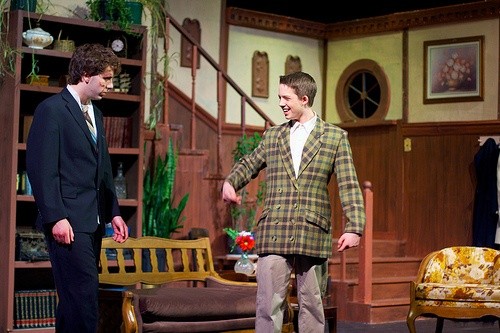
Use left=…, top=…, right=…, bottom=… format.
left=81, top=104, right=96, bottom=142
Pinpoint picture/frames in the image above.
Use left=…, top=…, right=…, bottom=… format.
left=423, top=35, right=485, bottom=104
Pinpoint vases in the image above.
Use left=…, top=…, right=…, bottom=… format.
left=220, top=252, right=260, bottom=279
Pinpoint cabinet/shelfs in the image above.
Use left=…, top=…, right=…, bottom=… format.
left=0, top=11, right=148, bottom=333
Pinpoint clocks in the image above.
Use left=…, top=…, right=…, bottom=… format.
left=107, top=33, right=127, bottom=58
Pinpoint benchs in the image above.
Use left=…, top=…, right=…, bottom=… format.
left=99, top=236, right=295, bottom=333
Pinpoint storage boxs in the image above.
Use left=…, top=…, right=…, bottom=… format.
left=16, top=233, right=50, bottom=261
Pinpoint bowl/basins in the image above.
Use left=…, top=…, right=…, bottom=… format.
left=55, top=39, right=75, bottom=53
left=22, top=27, right=54, bottom=50
left=25, top=74, right=49, bottom=86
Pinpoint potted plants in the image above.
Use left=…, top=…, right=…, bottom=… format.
left=135, top=136, right=192, bottom=271
left=0, top=0, right=56, bottom=82
left=85, top=0, right=150, bottom=37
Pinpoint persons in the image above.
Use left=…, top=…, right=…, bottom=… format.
left=25, top=43, right=129, bottom=333
left=222, top=71, right=366, bottom=333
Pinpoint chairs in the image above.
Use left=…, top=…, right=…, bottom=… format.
left=407, top=247, right=500, bottom=333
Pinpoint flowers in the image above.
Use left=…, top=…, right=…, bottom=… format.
left=225, top=133, right=265, bottom=252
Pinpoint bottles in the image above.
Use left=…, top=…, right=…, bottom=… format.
left=114, top=161, right=127, bottom=199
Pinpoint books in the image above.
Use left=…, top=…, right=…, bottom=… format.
left=23, top=116, right=130, bottom=148
left=14, top=289, right=57, bottom=328
